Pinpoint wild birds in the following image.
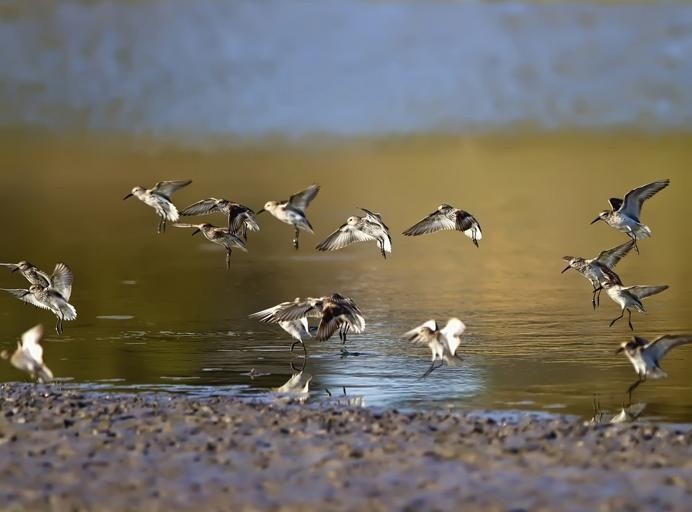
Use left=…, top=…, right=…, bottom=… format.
left=312, top=206, right=394, bottom=265
left=122, top=176, right=193, bottom=236
left=399, top=203, right=483, bottom=249
left=0, top=259, right=77, bottom=337
left=173, top=223, right=249, bottom=273
left=589, top=176, right=670, bottom=256
left=0, top=260, right=49, bottom=284
left=248, top=296, right=303, bottom=347
left=256, top=183, right=322, bottom=250
left=301, top=292, right=367, bottom=342
left=177, top=197, right=261, bottom=241
left=608, top=334, right=692, bottom=406
left=402, top=317, right=467, bottom=381
left=559, top=239, right=634, bottom=312
left=593, top=281, right=669, bottom=332
